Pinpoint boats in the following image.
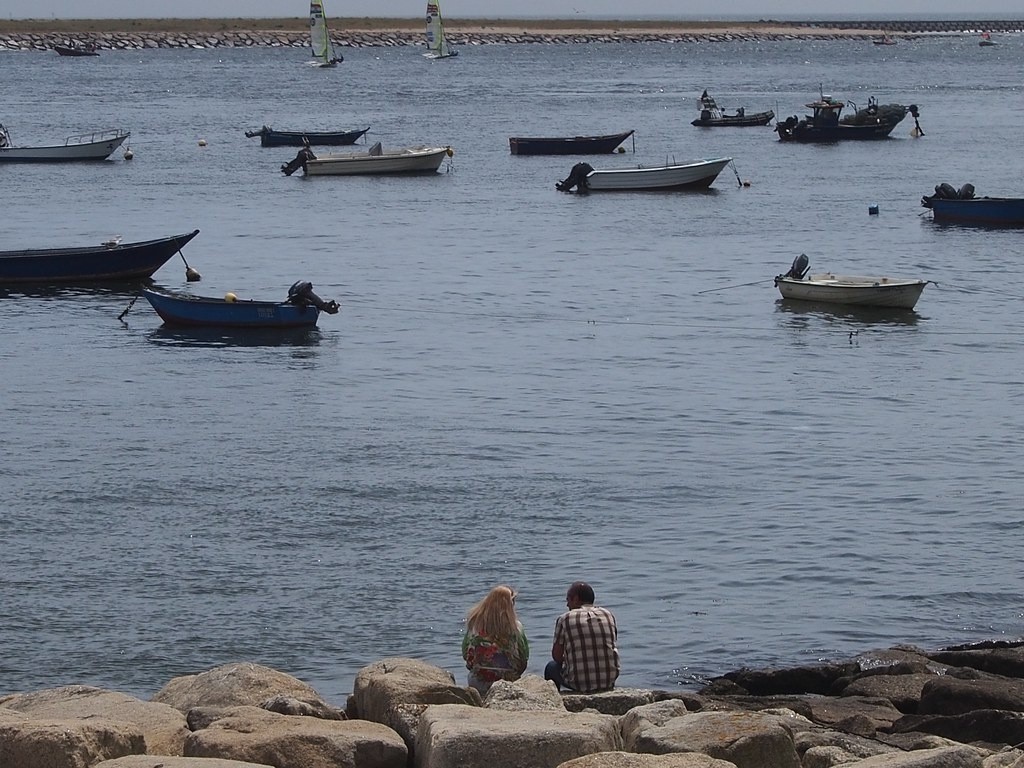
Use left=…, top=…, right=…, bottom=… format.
left=0, top=123, right=131, bottom=161
left=0, top=229, right=200, bottom=283
left=774, top=273, right=929, bottom=309
left=774, top=103, right=908, bottom=141
left=979, top=39, right=998, bottom=47
left=921, top=195, right=1024, bottom=223
left=54, top=46, right=100, bottom=56
left=580, top=156, right=733, bottom=192
left=138, top=279, right=323, bottom=327
left=691, top=107, right=774, bottom=127
left=873, top=40, right=897, bottom=45
left=305, top=141, right=450, bottom=175
left=260, top=125, right=370, bottom=146
left=508, top=129, right=636, bottom=156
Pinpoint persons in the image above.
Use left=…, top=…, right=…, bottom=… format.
left=68, top=40, right=98, bottom=52
left=719, top=107, right=725, bottom=118
left=830, top=99, right=839, bottom=105
left=700, top=106, right=711, bottom=120
left=544, top=581, right=620, bottom=692
left=461, top=585, right=529, bottom=696
left=882, top=33, right=899, bottom=43
left=786, top=114, right=797, bottom=132
left=981, top=30, right=992, bottom=41
left=735, top=108, right=741, bottom=117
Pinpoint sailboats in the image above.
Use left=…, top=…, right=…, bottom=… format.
left=305, top=0, right=338, bottom=68
left=422, top=0, right=450, bottom=60
